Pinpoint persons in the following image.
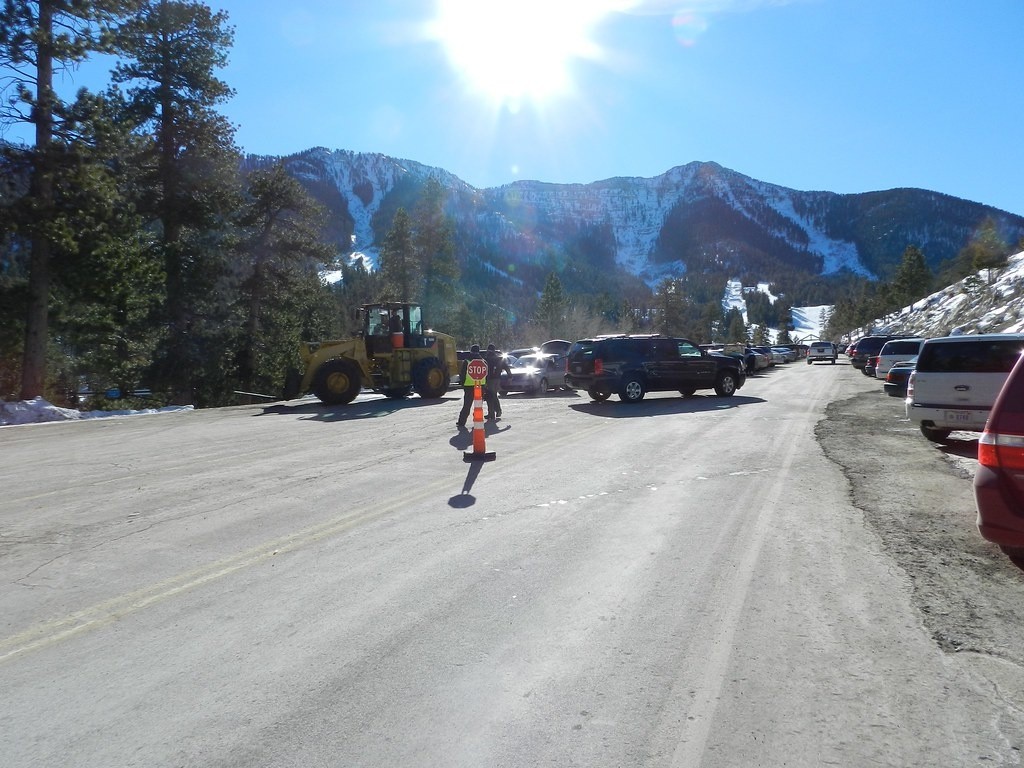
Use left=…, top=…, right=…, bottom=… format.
left=388, top=310, right=401, bottom=331
left=483, top=344, right=513, bottom=419
left=456, top=344, right=495, bottom=426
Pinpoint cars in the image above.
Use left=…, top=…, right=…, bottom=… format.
left=884, top=355, right=920, bottom=398
left=452, top=339, right=578, bottom=397
left=699, top=344, right=807, bottom=377
left=838, top=343, right=856, bottom=356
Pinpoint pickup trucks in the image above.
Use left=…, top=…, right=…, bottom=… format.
left=865, top=355, right=877, bottom=374
left=806, top=340, right=836, bottom=365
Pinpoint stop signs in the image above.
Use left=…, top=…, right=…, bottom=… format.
left=467, top=359, right=487, bottom=380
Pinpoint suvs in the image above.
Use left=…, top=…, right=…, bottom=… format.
left=905, top=333, right=1024, bottom=440
left=974, top=350, right=1024, bottom=573
left=565, top=334, right=745, bottom=403
left=875, top=338, right=926, bottom=379
left=850, top=335, right=921, bottom=376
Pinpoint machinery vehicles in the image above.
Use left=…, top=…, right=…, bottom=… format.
left=282, top=301, right=458, bottom=406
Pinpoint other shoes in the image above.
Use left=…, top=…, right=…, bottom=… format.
left=484, top=415, right=495, bottom=420
left=496, top=412, right=502, bottom=417
left=456, top=422, right=465, bottom=425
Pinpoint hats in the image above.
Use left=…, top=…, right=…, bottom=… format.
left=471, top=346, right=479, bottom=352
left=488, top=344, right=495, bottom=350
